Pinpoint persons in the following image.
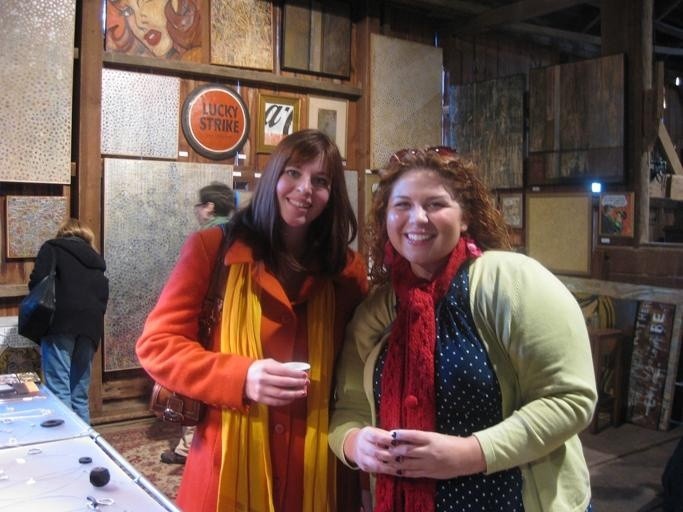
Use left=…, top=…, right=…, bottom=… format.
left=608, top=210, right=626, bottom=234
left=158, top=180, right=236, bottom=467
left=27, top=217, right=110, bottom=428
left=135, top=127, right=372, bottom=512
left=327, top=145, right=599, bottom=512
left=603, top=204, right=614, bottom=232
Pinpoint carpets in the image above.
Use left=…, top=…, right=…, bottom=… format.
left=100, top=412, right=683, bottom=512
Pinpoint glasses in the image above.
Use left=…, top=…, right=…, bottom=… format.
left=386, top=145, right=461, bottom=167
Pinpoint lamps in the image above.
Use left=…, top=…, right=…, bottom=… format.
left=591, top=182, right=602, bottom=199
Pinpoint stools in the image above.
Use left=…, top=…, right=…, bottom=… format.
left=589, top=328, right=624, bottom=434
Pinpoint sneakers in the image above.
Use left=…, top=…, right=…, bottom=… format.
left=159, top=447, right=187, bottom=464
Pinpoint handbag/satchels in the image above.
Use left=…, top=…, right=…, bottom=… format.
left=16, top=269, right=58, bottom=346
left=146, top=326, right=215, bottom=426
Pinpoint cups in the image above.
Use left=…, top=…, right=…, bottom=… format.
left=283, top=362, right=311, bottom=398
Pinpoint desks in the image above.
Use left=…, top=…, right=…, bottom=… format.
left=0, top=382, right=181, bottom=512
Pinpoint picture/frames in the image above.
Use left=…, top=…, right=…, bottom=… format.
left=306, top=93, right=349, bottom=161
left=490, top=191, right=635, bottom=277
left=256, top=94, right=302, bottom=154
